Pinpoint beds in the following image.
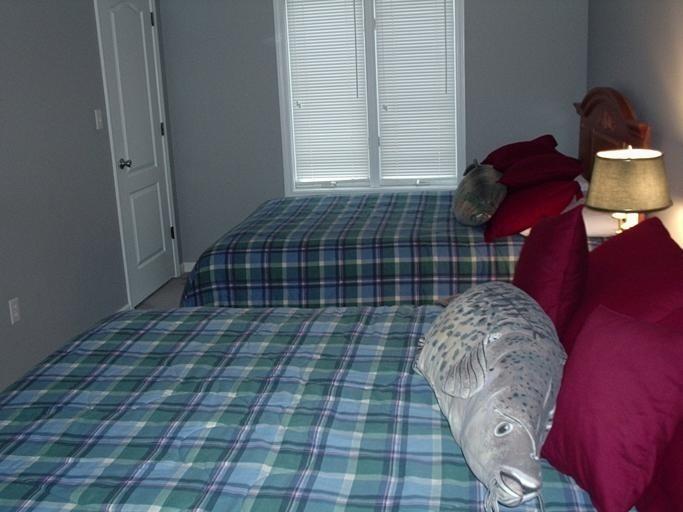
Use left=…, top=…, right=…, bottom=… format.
left=0, top=308, right=637, bottom=512
left=181, top=86, right=651, bottom=305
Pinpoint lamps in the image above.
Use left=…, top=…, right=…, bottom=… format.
left=586, top=147, right=673, bottom=234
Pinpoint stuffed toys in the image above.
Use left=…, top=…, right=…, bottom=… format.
left=414, top=276, right=569, bottom=512
left=450, top=156, right=507, bottom=227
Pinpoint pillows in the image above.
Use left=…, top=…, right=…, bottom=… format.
left=588, top=217, right=683, bottom=324
left=541, top=304, right=682, bottom=512
left=481, top=134, right=591, bottom=242
left=514, top=207, right=588, bottom=327
